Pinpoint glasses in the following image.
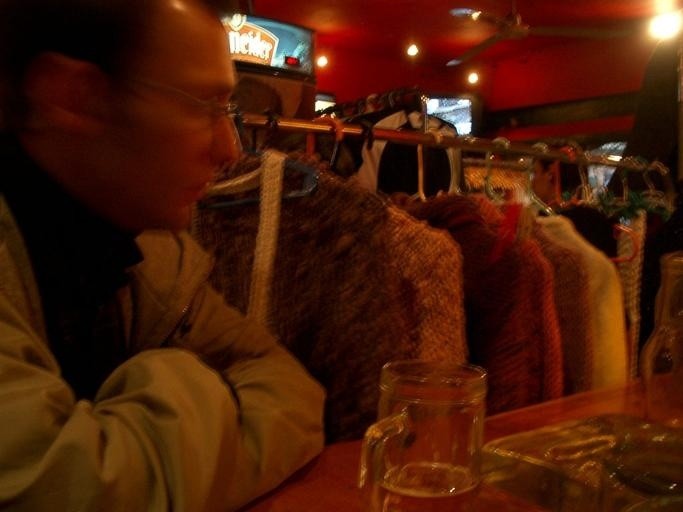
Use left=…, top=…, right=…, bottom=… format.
left=145, top=81, right=239, bottom=118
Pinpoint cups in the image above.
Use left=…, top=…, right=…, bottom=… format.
left=357, top=358, right=488, bottom=512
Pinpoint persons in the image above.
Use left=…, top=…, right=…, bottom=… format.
left=1, top=0, right=329, bottom=512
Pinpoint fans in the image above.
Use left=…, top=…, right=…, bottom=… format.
left=445, top=0, right=636, bottom=66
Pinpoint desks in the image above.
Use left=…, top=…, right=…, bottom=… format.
left=246, top=371, right=683, bottom=512
